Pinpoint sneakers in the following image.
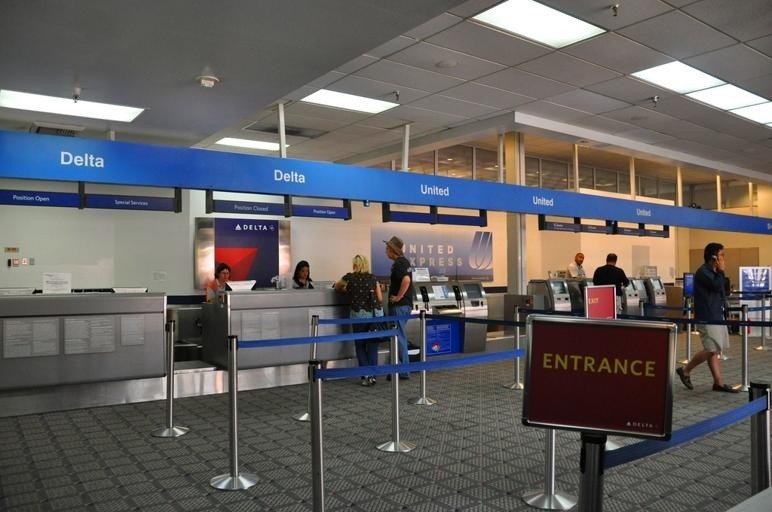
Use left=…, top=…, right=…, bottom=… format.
left=368, top=376, right=377, bottom=384
left=360, top=374, right=369, bottom=386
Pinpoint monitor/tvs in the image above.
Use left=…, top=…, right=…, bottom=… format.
left=225, top=280, right=257, bottom=291
left=652, top=279, right=663, bottom=290
left=110, top=287, right=149, bottom=294
left=463, top=284, right=483, bottom=299
left=0, top=287, right=44, bottom=296
left=307, top=280, right=336, bottom=290
left=552, top=281, right=566, bottom=294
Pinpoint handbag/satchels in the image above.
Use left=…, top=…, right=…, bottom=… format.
left=368, top=319, right=391, bottom=342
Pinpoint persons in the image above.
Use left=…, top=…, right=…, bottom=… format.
left=204, top=262, right=232, bottom=303
left=591, top=253, right=630, bottom=321
left=291, top=259, right=316, bottom=290
left=674, top=240, right=742, bottom=396
left=565, top=253, right=588, bottom=279
left=333, top=254, right=383, bottom=387
left=382, top=235, right=415, bottom=382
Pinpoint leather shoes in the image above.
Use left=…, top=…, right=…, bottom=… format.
left=712, top=383, right=738, bottom=393
left=676, top=367, right=693, bottom=390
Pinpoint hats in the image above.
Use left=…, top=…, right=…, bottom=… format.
left=382, top=235, right=403, bottom=257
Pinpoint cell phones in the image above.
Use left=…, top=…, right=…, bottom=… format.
left=711, top=255, right=717, bottom=263
